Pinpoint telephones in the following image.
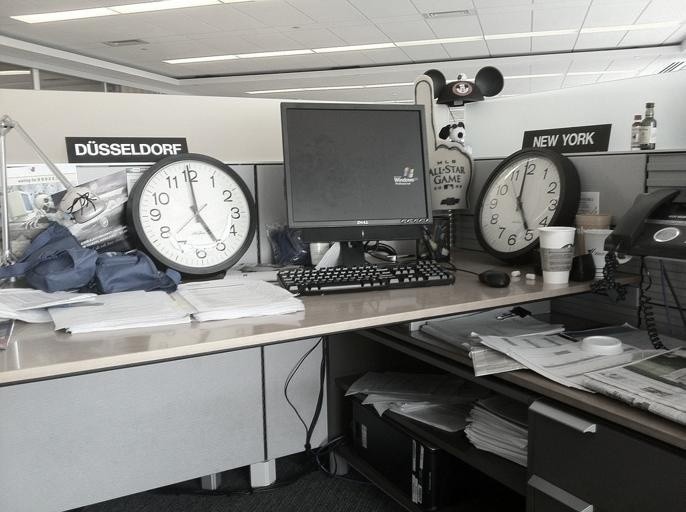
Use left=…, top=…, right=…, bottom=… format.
left=589, top=186, right=686, bottom=304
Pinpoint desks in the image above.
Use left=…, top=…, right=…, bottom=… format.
left=327, top=327, right=685, bottom=512
left=0, top=89, right=647, bottom=511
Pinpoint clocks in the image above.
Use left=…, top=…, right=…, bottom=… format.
left=127, top=152, right=259, bottom=277
left=472, top=148, right=580, bottom=265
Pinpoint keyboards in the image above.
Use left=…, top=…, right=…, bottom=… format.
left=277, top=263, right=455, bottom=296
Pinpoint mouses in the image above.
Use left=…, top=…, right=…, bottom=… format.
left=478, top=269, right=510, bottom=287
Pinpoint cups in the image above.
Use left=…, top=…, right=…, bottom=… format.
left=538, top=226, right=575, bottom=284
left=585, top=229, right=614, bottom=278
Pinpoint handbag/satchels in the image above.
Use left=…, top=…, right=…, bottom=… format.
left=95, top=249, right=181, bottom=294
left=0, top=221, right=99, bottom=293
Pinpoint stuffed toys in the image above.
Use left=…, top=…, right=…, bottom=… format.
left=439, top=122, right=473, bottom=157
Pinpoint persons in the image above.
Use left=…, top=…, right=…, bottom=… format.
left=24, top=189, right=69, bottom=230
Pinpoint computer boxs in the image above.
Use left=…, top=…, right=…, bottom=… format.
left=347, top=401, right=436, bottom=512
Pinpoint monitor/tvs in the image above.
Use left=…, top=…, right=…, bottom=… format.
left=280, top=101, right=434, bottom=264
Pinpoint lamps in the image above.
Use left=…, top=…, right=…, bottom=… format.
left=428, top=9, right=471, bottom=20
left=101, top=38, right=152, bottom=48
left=0, top=114, right=108, bottom=273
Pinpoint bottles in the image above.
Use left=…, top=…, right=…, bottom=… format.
left=640, top=103, right=657, bottom=150
left=630, top=115, right=642, bottom=150
